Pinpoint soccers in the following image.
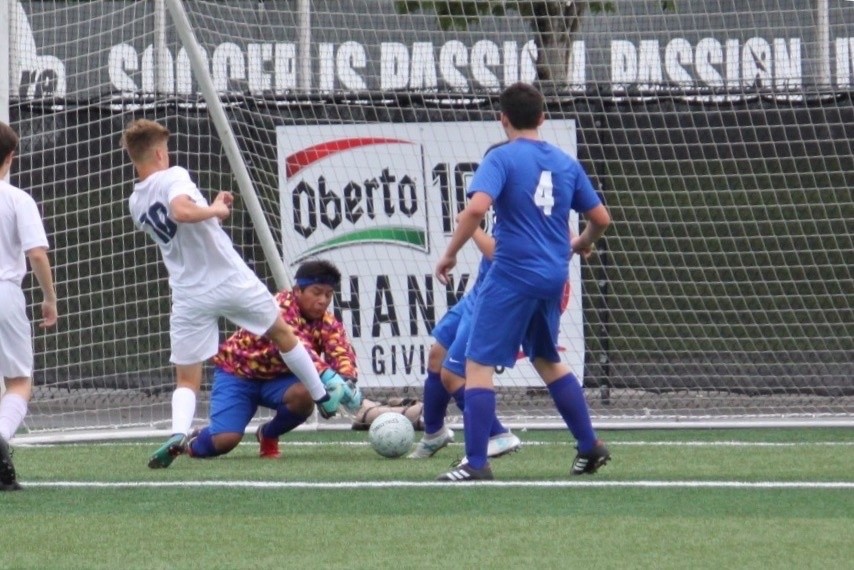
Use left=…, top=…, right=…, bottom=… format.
left=367, top=411, right=416, bottom=458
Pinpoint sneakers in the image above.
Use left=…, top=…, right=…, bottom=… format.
left=316, top=384, right=346, bottom=419
left=433, top=455, right=495, bottom=479
left=148, top=433, right=189, bottom=469
left=0, top=432, right=22, bottom=491
left=406, top=424, right=455, bottom=458
left=569, top=440, right=613, bottom=474
left=256, top=422, right=283, bottom=459
left=487, top=429, right=522, bottom=456
left=186, top=427, right=201, bottom=457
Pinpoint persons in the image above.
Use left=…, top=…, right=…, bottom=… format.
left=407, top=210, right=522, bottom=462
left=0, top=121, right=58, bottom=492
left=435, top=81, right=613, bottom=483
left=120, top=118, right=345, bottom=469
left=185, top=257, right=358, bottom=459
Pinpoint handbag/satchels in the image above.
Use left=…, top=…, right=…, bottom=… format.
left=351, top=398, right=425, bottom=430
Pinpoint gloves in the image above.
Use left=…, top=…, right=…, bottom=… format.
left=320, top=367, right=356, bottom=404
left=341, top=375, right=364, bottom=412
